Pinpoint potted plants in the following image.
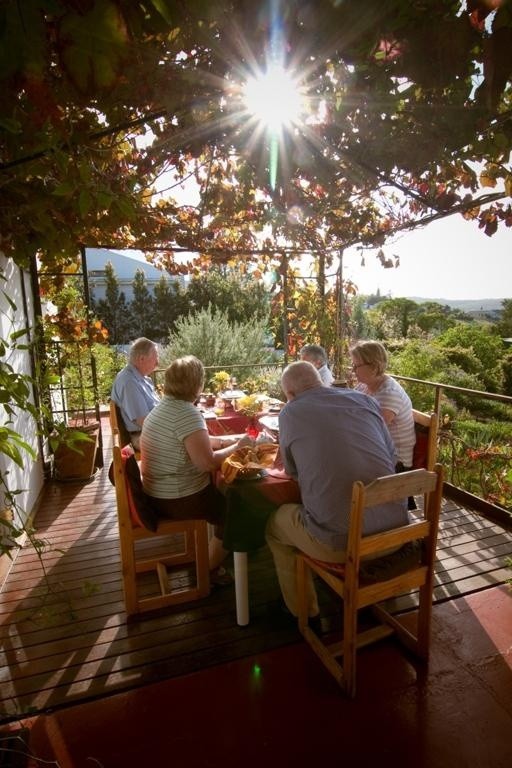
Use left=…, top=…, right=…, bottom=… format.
left=29, top=340, right=102, bottom=486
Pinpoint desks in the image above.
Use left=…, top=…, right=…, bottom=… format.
left=186, top=389, right=300, bottom=627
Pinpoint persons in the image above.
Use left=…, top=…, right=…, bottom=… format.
left=348, top=340, right=417, bottom=474
left=286, top=344, right=335, bottom=403
left=110, top=336, right=161, bottom=451
left=139, top=355, right=257, bottom=588
left=261, top=360, right=410, bottom=632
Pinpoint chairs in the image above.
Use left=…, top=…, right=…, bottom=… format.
left=110, top=426, right=213, bottom=618
left=402, top=404, right=440, bottom=535
left=110, top=400, right=152, bottom=463
left=292, top=461, right=441, bottom=697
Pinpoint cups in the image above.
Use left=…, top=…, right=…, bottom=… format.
left=214, top=401, right=225, bottom=416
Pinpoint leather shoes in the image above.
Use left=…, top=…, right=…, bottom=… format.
left=267, top=597, right=329, bottom=635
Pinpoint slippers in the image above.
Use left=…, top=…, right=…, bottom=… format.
left=193, top=566, right=234, bottom=586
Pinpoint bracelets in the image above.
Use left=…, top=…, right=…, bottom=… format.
left=221, top=439, right=223, bottom=448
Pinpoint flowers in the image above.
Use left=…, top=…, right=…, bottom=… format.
left=208, top=371, right=235, bottom=393
